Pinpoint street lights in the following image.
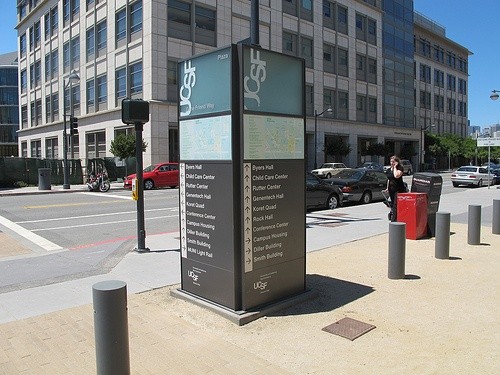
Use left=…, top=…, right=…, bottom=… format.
left=315, top=107, right=333, bottom=169
left=420, top=123, right=436, bottom=170
left=63, top=70, right=80, bottom=189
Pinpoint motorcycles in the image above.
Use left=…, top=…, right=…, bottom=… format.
left=86, top=170, right=111, bottom=193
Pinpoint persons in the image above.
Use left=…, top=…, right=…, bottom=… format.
left=385, top=155, right=405, bottom=222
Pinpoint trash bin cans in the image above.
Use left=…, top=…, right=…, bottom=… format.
left=396, top=191, right=428, bottom=240
left=410, top=171, right=442, bottom=237
left=38, top=168, right=51, bottom=190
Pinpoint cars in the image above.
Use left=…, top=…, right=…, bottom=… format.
left=383, top=159, right=413, bottom=176
left=123, top=163, right=180, bottom=190
left=305, top=171, right=344, bottom=211
left=323, top=168, right=409, bottom=204
left=312, top=162, right=353, bottom=179
left=452, top=165, right=497, bottom=187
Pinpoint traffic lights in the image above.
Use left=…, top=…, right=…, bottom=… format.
left=71, top=117, right=78, bottom=134
